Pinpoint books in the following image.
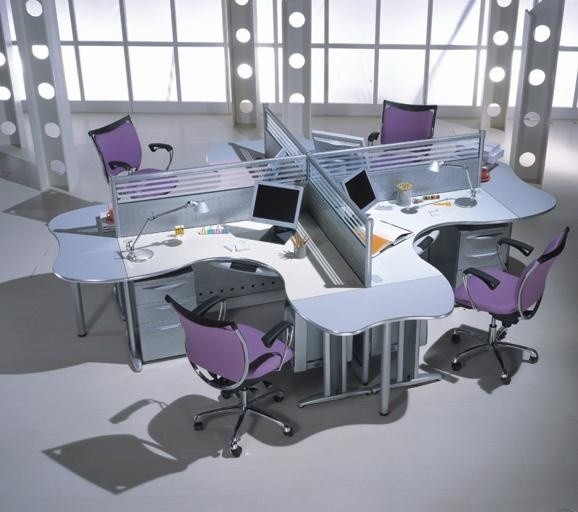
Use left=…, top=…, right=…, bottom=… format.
left=353, top=217, right=416, bottom=259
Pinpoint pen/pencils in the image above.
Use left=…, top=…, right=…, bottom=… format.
left=223, top=246, right=237, bottom=252
left=291, top=232, right=310, bottom=246
left=395, top=183, right=412, bottom=191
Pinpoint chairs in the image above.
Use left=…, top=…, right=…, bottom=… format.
left=88, top=115, right=179, bottom=200
left=451, top=225, right=569, bottom=383
left=164, top=295, right=294, bottom=458
left=367, top=100, right=438, bottom=170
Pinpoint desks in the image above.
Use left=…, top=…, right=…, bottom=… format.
left=47, top=136, right=559, bottom=416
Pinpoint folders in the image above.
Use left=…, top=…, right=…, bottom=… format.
left=445, top=139, right=505, bottom=168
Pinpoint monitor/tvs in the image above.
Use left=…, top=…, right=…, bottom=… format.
left=340, top=167, right=379, bottom=219
left=249, top=178, right=305, bottom=244
left=311, top=129, right=370, bottom=170
left=261, top=146, right=284, bottom=183
